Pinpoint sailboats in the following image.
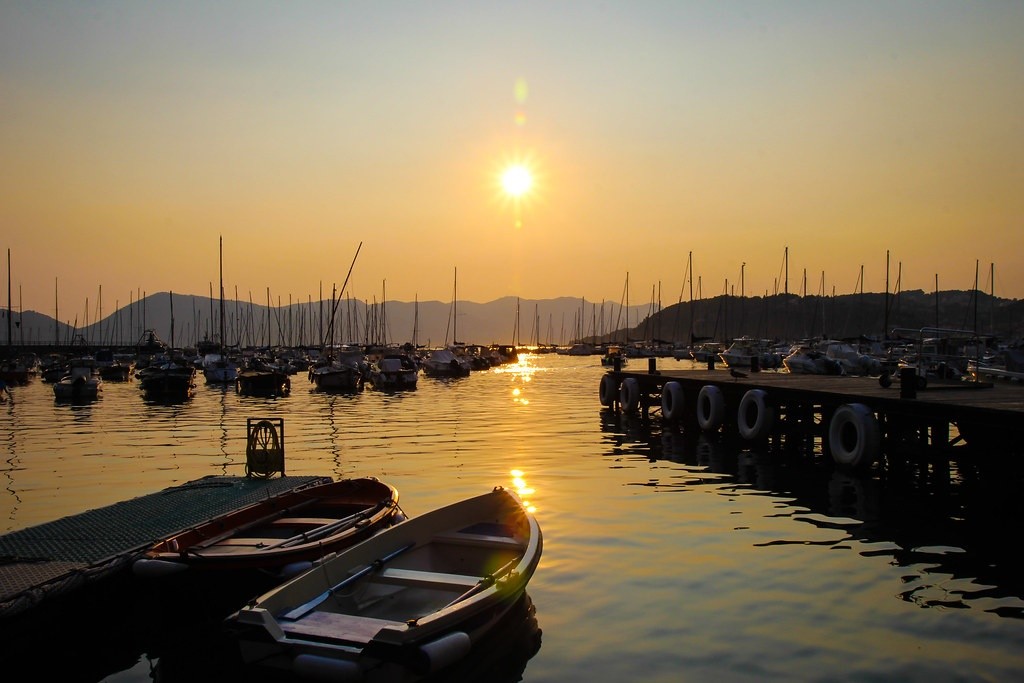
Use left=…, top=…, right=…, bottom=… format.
left=0, top=235, right=556, bottom=391
left=556, top=247, right=1024, bottom=382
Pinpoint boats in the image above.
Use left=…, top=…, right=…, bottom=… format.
left=53, top=368, right=102, bottom=404
left=140, top=359, right=195, bottom=400
left=223, top=487, right=543, bottom=683
left=235, top=358, right=286, bottom=396
left=142, top=475, right=399, bottom=573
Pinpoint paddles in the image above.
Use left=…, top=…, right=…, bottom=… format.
left=286, top=542, right=418, bottom=622
left=269, top=502, right=384, bottom=549
left=198, top=493, right=324, bottom=547
left=441, top=556, right=521, bottom=607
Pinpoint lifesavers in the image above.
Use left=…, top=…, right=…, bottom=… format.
left=599, top=373, right=615, bottom=404
left=829, top=402, right=876, bottom=464
left=661, top=380, right=682, bottom=421
left=738, top=388, right=772, bottom=437
left=695, top=386, right=723, bottom=429
left=619, top=378, right=637, bottom=411
